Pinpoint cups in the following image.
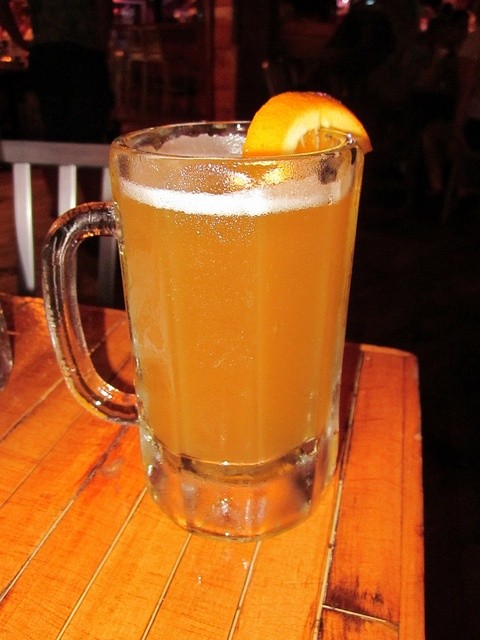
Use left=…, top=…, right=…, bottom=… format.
left=39, top=119, right=367, bottom=539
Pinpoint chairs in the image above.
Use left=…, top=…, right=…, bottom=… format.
left=0, top=139, right=116, bottom=311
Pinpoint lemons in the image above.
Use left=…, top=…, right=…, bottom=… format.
left=241, top=87, right=374, bottom=182
